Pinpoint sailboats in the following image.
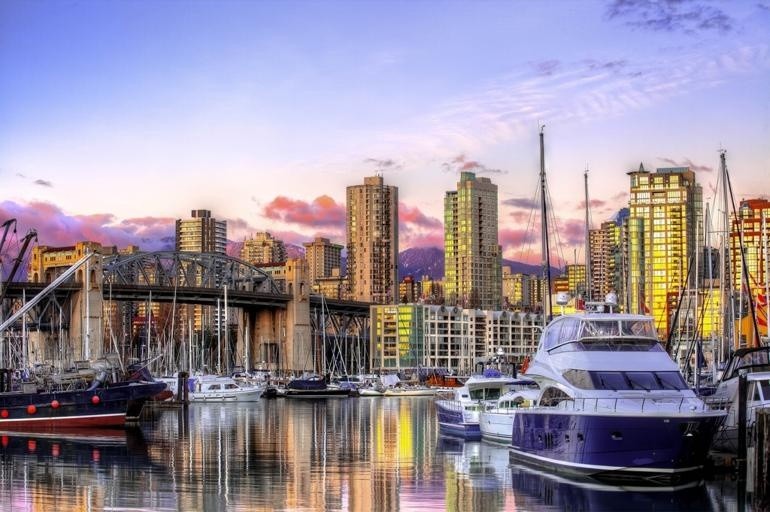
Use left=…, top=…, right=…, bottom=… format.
left=662, top=148, right=770, bottom=452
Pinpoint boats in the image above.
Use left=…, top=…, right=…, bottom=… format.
left=0, top=428, right=150, bottom=469
left=438, top=435, right=717, bottom=512
left=0, top=364, right=165, bottom=427
